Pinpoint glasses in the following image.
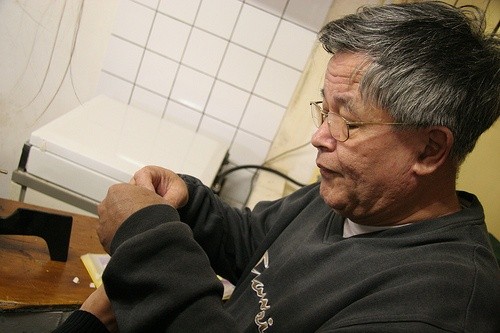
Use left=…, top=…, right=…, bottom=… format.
left=310, top=101, right=415, bottom=142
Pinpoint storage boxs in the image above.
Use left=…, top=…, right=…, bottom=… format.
left=11, top=93, right=230, bottom=219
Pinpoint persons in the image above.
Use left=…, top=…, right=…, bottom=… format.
left=53, top=280, right=115, bottom=333
left=97, top=0, right=500, bottom=333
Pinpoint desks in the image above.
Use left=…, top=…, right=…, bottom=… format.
left=0, top=197, right=106, bottom=311
